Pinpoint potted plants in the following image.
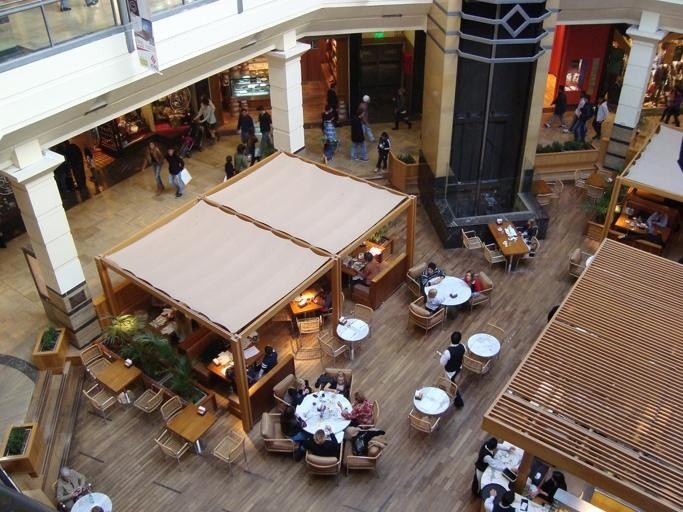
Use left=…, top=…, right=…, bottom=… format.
left=32, top=324, right=70, bottom=375
left=533, top=137, right=599, bottom=174
left=576, top=176, right=628, bottom=242
left=368, top=225, right=394, bottom=262
left=394, top=149, right=418, bottom=165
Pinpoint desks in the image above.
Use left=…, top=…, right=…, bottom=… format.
left=585, top=255, right=594, bottom=268
left=70, top=492, right=112, bottom=512
left=340, top=241, right=386, bottom=289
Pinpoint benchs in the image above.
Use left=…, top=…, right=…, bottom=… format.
left=615, top=187, right=681, bottom=257
left=351, top=252, right=408, bottom=311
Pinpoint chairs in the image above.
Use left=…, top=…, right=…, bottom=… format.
left=50, top=481, right=66, bottom=512
left=407, top=219, right=541, bottom=438
left=568, top=244, right=595, bottom=279
left=530, top=167, right=615, bottom=207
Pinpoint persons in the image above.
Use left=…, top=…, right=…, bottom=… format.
left=317, top=81, right=409, bottom=171
left=51, top=140, right=96, bottom=194
left=419, top=261, right=445, bottom=303
left=536, top=470, right=567, bottom=505
left=545, top=84, right=610, bottom=144
left=661, top=84, right=682, bottom=128
left=646, top=207, right=669, bottom=235
left=336, top=390, right=376, bottom=428
left=141, top=293, right=278, bottom=396
left=439, top=331, right=467, bottom=413
left=517, top=218, right=537, bottom=245
left=311, top=283, right=332, bottom=317
left=323, top=369, right=348, bottom=398
left=279, top=406, right=307, bottom=462
left=350, top=252, right=380, bottom=293
left=141, top=95, right=273, bottom=198
left=482, top=486, right=516, bottom=511
left=282, top=377, right=313, bottom=404
left=470, top=435, right=518, bottom=498
left=55, top=466, right=91, bottom=511
left=307, top=425, right=338, bottom=457
left=463, top=271, right=481, bottom=298
left=57, top=0, right=97, bottom=12
left=425, top=289, right=446, bottom=313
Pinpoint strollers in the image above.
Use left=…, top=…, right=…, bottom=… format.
left=176, top=115, right=202, bottom=163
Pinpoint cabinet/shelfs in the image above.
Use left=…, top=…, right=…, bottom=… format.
left=229, top=73, right=270, bottom=108
left=94, top=109, right=156, bottom=159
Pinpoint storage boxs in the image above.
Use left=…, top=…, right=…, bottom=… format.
left=0, top=423, right=45, bottom=473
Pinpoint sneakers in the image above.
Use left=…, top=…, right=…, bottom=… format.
left=351, top=156, right=369, bottom=161
left=563, top=129, right=600, bottom=143
left=544, top=122, right=552, bottom=128
left=408, top=123, right=413, bottom=129
left=323, top=155, right=334, bottom=165
left=374, top=167, right=387, bottom=172
left=176, top=191, right=183, bottom=199
left=559, top=124, right=566, bottom=127
left=391, top=126, right=400, bottom=130
left=157, top=186, right=164, bottom=194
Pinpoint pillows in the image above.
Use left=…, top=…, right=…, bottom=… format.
left=570, top=247, right=581, bottom=265
